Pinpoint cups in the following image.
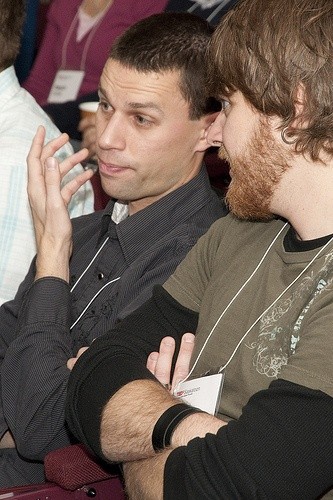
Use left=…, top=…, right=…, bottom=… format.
left=78, top=102, right=100, bottom=121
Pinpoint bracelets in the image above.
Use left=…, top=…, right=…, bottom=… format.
left=150, top=402, right=210, bottom=458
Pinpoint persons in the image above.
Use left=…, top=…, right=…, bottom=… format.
left=22, top=0, right=171, bottom=115
left=0, top=0, right=98, bottom=313
left=0, top=9, right=231, bottom=494
left=64, top=0, right=333, bottom=500
